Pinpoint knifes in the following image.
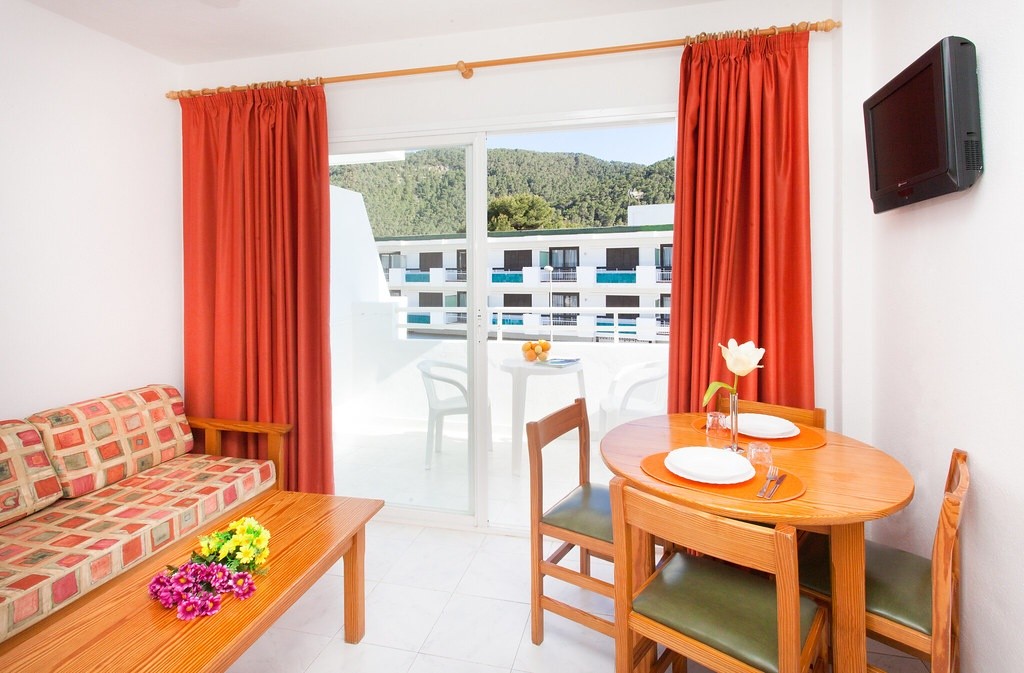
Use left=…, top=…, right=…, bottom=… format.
left=764, top=474, right=787, bottom=500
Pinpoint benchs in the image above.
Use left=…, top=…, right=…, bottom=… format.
left=0, top=382, right=293, bottom=648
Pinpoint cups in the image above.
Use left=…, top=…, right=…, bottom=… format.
left=748, top=441, right=772, bottom=470
left=706, top=411, right=728, bottom=437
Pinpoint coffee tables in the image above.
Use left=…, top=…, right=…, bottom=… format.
left=0, top=493, right=385, bottom=673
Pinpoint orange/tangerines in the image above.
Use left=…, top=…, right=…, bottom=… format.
left=522, top=339, right=551, bottom=361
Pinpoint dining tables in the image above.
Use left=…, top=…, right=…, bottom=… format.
left=600, top=413, right=915, bottom=673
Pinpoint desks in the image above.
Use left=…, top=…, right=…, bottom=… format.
left=504, top=350, right=591, bottom=473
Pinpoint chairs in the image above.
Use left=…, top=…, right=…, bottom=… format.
left=594, top=353, right=671, bottom=430
left=769, top=451, right=972, bottom=672
left=524, top=398, right=655, bottom=672
left=606, top=478, right=828, bottom=673
left=416, top=356, right=500, bottom=468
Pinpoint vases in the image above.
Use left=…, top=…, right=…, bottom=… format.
left=722, top=393, right=746, bottom=454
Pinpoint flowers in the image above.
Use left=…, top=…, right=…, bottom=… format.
left=149, top=513, right=270, bottom=619
left=702, top=336, right=766, bottom=424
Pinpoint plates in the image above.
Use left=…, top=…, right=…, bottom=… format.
left=664, top=447, right=755, bottom=485
left=720, top=413, right=800, bottom=439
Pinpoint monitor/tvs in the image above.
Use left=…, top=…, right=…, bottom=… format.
left=863, top=35, right=984, bottom=213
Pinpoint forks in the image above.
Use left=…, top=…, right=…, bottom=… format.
left=757, top=466, right=779, bottom=497
